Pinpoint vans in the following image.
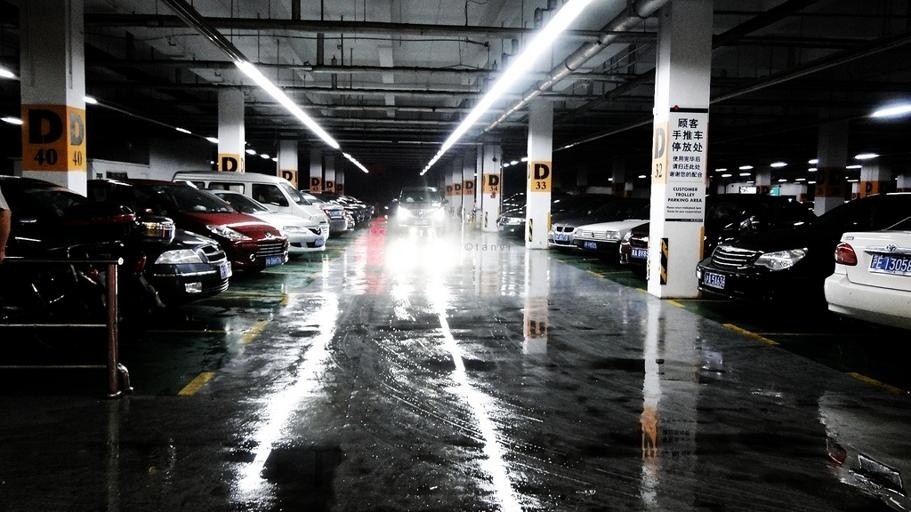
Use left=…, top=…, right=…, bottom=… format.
left=392, top=183, right=454, bottom=233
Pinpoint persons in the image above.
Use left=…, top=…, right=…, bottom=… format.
left=0, top=187, right=13, bottom=261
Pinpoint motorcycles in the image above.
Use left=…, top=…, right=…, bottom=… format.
left=0, top=202, right=178, bottom=373
